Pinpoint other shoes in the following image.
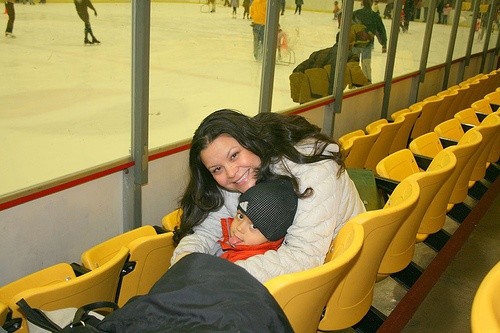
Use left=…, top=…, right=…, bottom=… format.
left=84, top=38, right=91, bottom=44
left=92, top=38, right=101, bottom=45
left=253, top=53, right=260, bottom=61
left=5, top=32, right=16, bottom=38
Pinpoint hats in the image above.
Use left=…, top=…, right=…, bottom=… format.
left=237, top=176, right=298, bottom=241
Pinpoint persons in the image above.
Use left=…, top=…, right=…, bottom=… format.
left=74, top=0, right=101, bottom=45
left=290, top=28, right=371, bottom=105
left=3, top=0, right=16, bottom=37
left=249, top=0, right=268, bottom=59
left=170, top=109, right=366, bottom=285
left=352, top=0, right=387, bottom=84
left=206, top=0, right=500, bottom=41
left=211, top=176, right=299, bottom=264
left=14, top=0, right=46, bottom=4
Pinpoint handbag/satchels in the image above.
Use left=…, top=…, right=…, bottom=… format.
left=57, top=302, right=123, bottom=332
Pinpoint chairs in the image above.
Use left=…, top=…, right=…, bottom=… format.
left=0, top=67, right=500, bottom=333
